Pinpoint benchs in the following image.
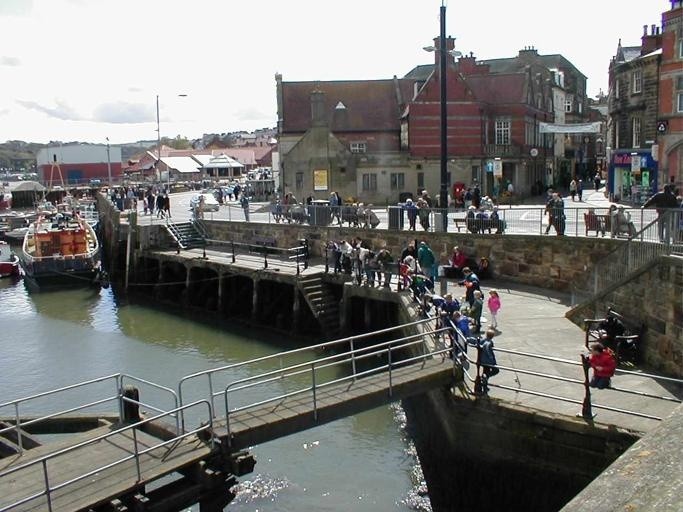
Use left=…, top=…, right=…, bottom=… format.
left=453, top=217, right=505, bottom=234
left=271, top=211, right=310, bottom=225
left=335, top=213, right=376, bottom=229
left=584, top=306, right=646, bottom=366
left=583, top=213, right=632, bottom=240
left=248, top=236, right=277, bottom=253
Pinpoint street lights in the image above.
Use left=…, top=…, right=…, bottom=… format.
left=423, top=0, right=464, bottom=231
left=103, top=135, right=112, bottom=192
left=154, top=92, right=189, bottom=194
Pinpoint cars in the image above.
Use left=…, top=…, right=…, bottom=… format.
left=189, top=194, right=218, bottom=212
left=203, top=166, right=272, bottom=200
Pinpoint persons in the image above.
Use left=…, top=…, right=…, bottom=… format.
left=259, top=172, right=268, bottom=181
left=586, top=344, right=616, bottom=390
left=111, top=185, right=137, bottom=221
left=327, top=239, right=500, bottom=391
left=569, top=179, right=577, bottom=202
left=271, top=192, right=315, bottom=225
left=543, top=188, right=557, bottom=235
left=404, top=190, right=433, bottom=232
left=143, top=192, right=171, bottom=218
left=217, top=185, right=249, bottom=221
left=576, top=180, right=585, bottom=202
left=185, top=179, right=206, bottom=190
left=330, top=191, right=380, bottom=229
left=452, top=179, right=513, bottom=234
left=587, top=183, right=683, bottom=244
left=594, top=173, right=601, bottom=192
left=544, top=192, right=566, bottom=234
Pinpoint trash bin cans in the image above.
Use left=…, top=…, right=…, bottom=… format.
left=454, top=182, right=465, bottom=198
left=117, top=199, right=125, bottom=212
left=389, top=206, right=404, bottom=229
left=310, top=199, right=330, bottom=225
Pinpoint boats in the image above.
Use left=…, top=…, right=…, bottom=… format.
left=0, top=155, right=105, bottom=285
